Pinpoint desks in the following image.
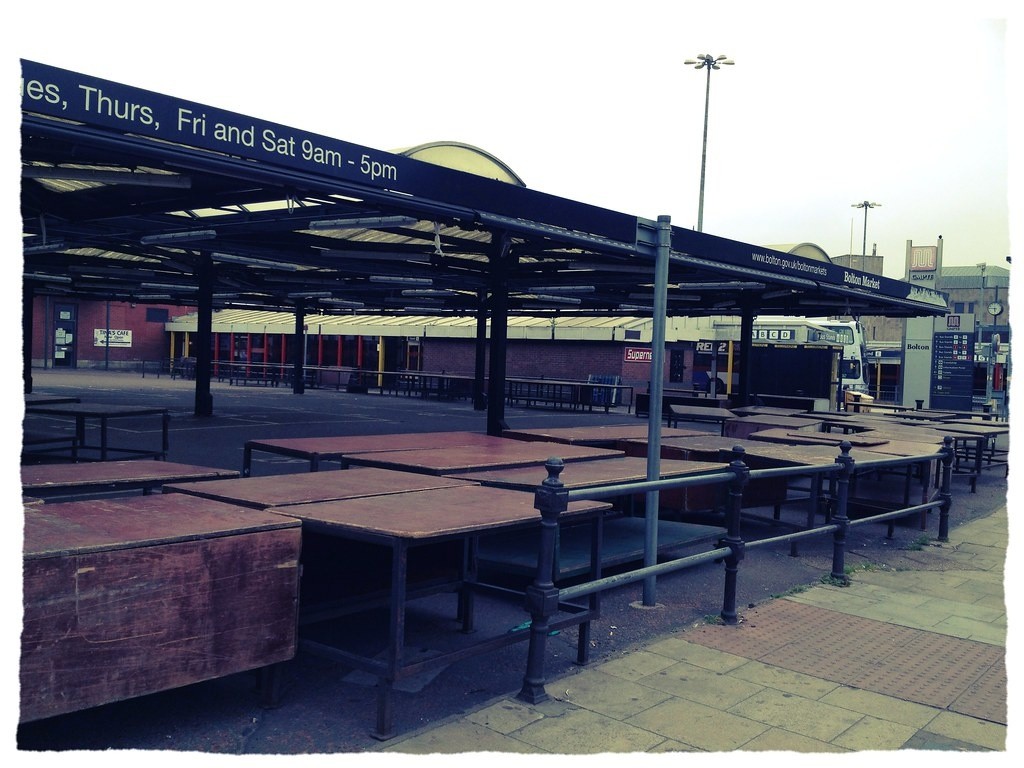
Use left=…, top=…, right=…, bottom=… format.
left=22, top=358, right=1008, bottom=733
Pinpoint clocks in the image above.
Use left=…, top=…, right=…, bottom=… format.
left=988, top=302, right=1004, bottom=316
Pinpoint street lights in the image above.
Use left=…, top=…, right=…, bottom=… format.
left=851, top=201, right=882, bottom=271
left=683, top=53, right=735, bottom=234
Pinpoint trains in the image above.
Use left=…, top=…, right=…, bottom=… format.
left=758, top=316, right=869, bottom=400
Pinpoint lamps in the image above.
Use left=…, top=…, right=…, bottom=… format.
left=22, top=165, right=869, bottom=313
